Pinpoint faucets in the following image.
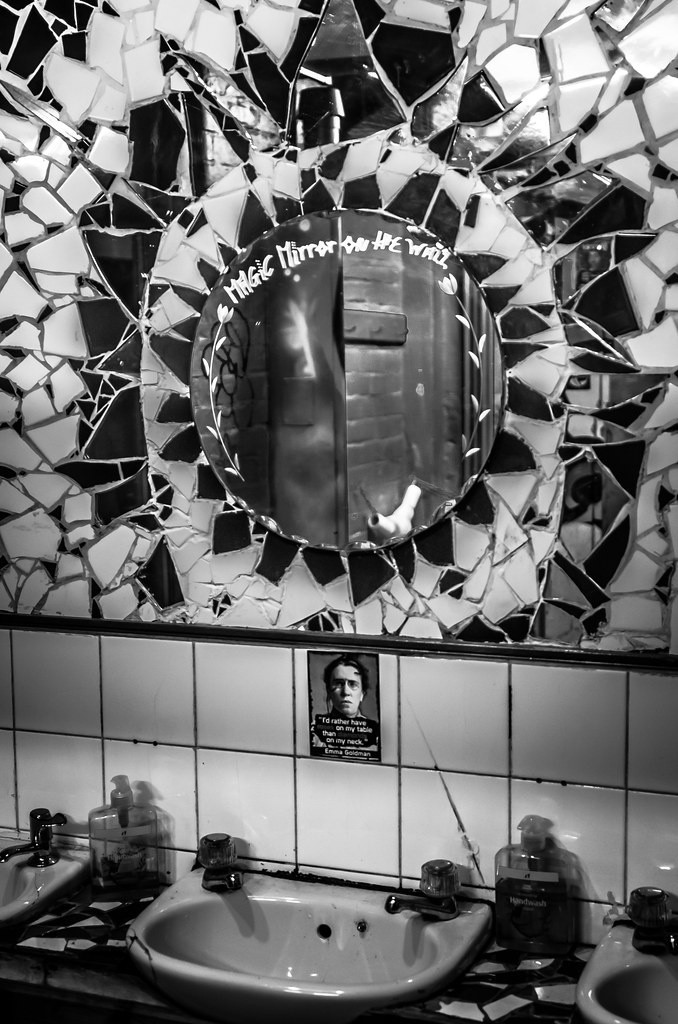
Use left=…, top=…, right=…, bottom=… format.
left=0, top=807, right=68, bottom=868
left=624, top=886, right=677, bottom=958
left=383, top=858, right=465, bottom=922
left=197, top=831, right=243, bottom=895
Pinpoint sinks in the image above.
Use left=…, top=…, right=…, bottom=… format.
left=574, top=924, right=678, bottom=1024
left=126, top=865, right=494, bottom=1012
left=0, top=833, right=91, bottom=923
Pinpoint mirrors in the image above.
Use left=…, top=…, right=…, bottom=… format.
left=188, top=209, right=515, bottom=559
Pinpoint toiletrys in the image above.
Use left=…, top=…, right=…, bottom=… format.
left=88, top=774, right=159, bottom=899
left=492, top=814, right=582, bottom=953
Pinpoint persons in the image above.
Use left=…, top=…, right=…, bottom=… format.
left=312, top=653, right=380, bottom=751
